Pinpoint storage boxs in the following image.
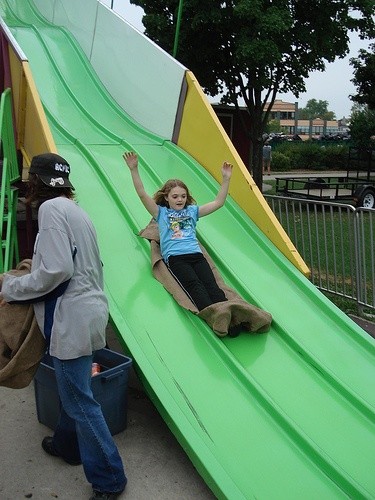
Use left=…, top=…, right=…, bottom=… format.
left=34, top=347, right=133, bottom=436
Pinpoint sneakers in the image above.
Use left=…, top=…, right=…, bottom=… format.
left=42, top=436, right=77, bottom=466
left=89, top=490, right=117, bottom=500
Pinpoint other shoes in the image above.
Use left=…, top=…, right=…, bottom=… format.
left=229, top=322, right=240, bottom=337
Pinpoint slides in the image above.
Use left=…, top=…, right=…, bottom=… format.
left=0, top=0, right=375, bottom=500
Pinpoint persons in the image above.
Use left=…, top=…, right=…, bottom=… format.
left=262, top=141, right=273, bottom=176
left=123, top=151, right=242, bottom=338
left=0, top=153, right=127, bottom=500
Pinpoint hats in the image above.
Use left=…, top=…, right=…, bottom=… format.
left=29, top=152, right=75, bottom=191
